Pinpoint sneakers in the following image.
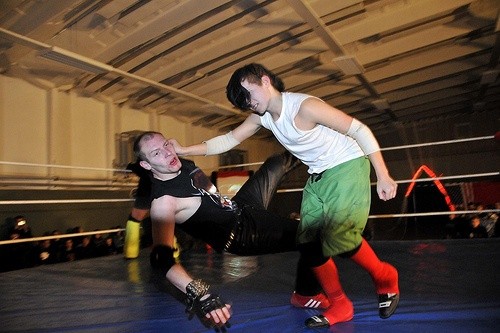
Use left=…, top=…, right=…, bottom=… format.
left=373, top=262, right=399, bottom=318
left=305, top=296, right=354, bottom=327
left=291, top=290, right=330, bottom=309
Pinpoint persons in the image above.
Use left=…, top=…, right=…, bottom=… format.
left=0, top=214, right=125, bottom=282
left=132, top=129, right=307, bottom=326
left=439, top=192, right=500, bottom=240
left=164, top=60, right=402, bottom=330
